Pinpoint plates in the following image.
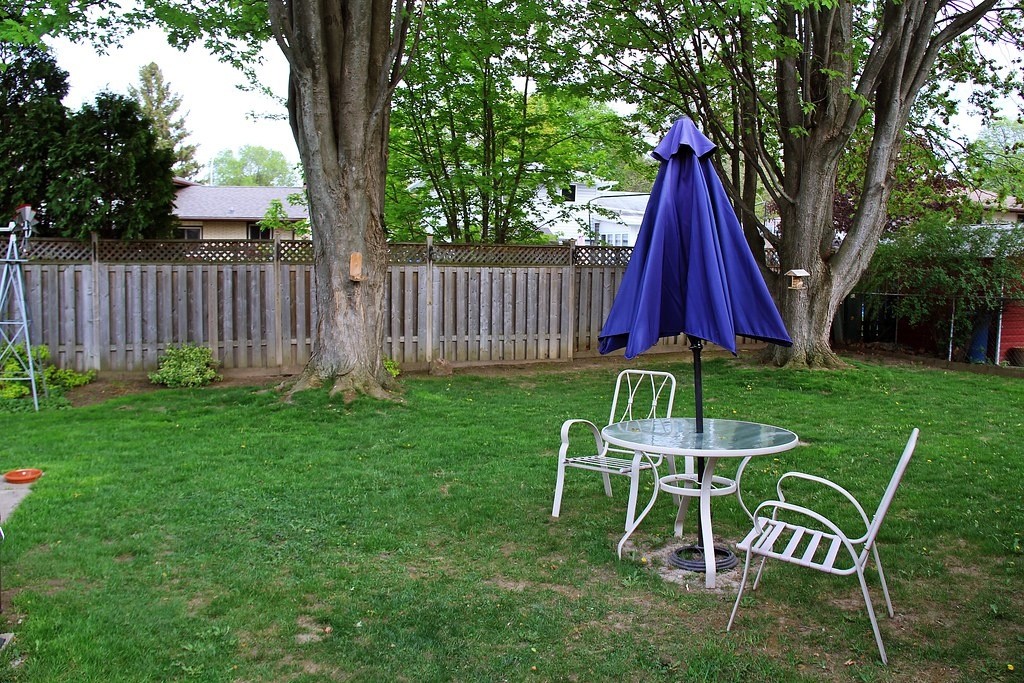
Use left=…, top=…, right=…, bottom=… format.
left=4, top=470, right=42, bottom=482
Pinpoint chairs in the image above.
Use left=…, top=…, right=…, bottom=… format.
left=552, top=369, right=676, bottom=532
left=725, top=428, right=920, bottom=666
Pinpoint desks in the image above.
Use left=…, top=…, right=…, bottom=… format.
left=601, top=417, right=798, bottom=589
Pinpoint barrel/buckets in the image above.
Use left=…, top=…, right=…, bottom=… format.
left=1008, top=347, right=1024, bottom=367
left=968, top=317, right=990, bottom=364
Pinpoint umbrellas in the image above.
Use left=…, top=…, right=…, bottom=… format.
left=597, top=115, right=793, bottom=571
